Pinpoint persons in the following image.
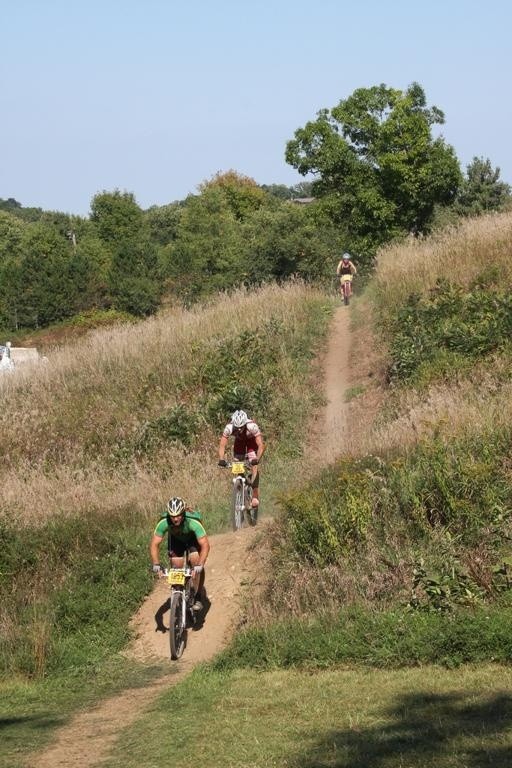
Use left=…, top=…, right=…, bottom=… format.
left=336, top=252, right=357, bottom=301
left=217, top=408, right=264, bottom=509
left=151, top=496, right=210, bottom=612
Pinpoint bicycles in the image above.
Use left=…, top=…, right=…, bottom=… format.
left=340, top=271, right=356, bottom=307
left=156, top=558, right=203, bottom=660
left=223, top=457, right=261, bottom=531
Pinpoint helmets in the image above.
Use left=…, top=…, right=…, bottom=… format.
left=342, top=253, right=350, bottom=259
left=167, top=498, right=185, bottom=516
left=230, top=410, right=247, bottom=427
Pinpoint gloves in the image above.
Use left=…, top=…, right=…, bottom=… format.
left=193, top=565, right=202, bottom=573
left=152, top=564, right=160, bottom=573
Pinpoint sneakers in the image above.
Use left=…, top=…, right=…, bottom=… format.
left=192, top=600, right=204, bottom=610
left=252, top=497, right=259, bottom=507
